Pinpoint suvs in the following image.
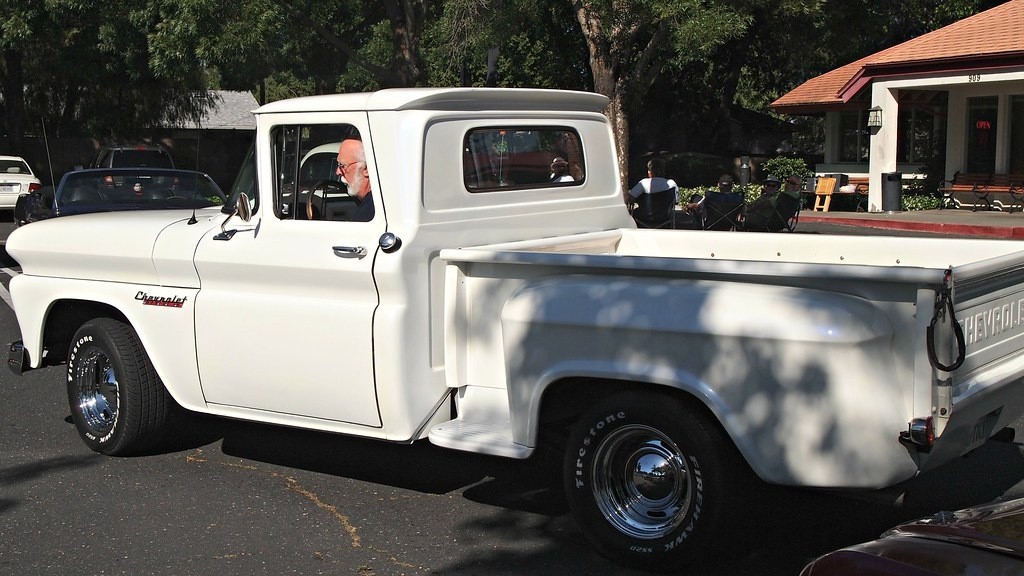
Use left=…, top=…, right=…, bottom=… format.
left=87, top=143, right=181, bottom=202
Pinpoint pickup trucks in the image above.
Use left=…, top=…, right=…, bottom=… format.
left=3, top=86, right=1024, bottom=576
left=13, top=168, right=228, bottom=230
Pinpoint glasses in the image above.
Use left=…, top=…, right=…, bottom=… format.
left=339, top=160, right=363, bottom=168
left=557, top=165, right=564, bottom=168
left=767, top=184, right=776, bottom=187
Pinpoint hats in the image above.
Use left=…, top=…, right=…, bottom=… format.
left=550, top=158, right=568, bottom=170
left=719, top=175, right=732, bottom=184
left=647, top=158, right=666, bottom=177
left=764, top=176, right=781, bottom=189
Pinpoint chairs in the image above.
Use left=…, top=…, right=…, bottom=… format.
left=634, top=187, right=803, bottom=232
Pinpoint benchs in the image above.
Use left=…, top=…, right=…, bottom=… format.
left=937, top=171, right=1024, bottom=214
left=803, top=177, right=869, bottom=212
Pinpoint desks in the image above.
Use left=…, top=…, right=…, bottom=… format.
left=814, top=178, right=836, bottom=212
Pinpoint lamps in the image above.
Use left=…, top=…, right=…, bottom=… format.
left=867, top=105, right=883, bottom=127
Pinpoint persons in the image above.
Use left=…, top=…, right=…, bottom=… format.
left=551, top=157, right=575, bottom=182
left=736, top=176, right=781, bottom=231
left=784, top=175, right=802, bottom=200
left=629, top=158, right=679, bottom=229
left=131, top=181, right=144, bottom=196
left=683, top=174, right=744, bottom=231
left=337, top=135, right=376, bottom=222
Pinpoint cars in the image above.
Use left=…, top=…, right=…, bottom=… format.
left=0, top=156, right=42, bottom=211
left=199, top=141, right=349, bottom=210
